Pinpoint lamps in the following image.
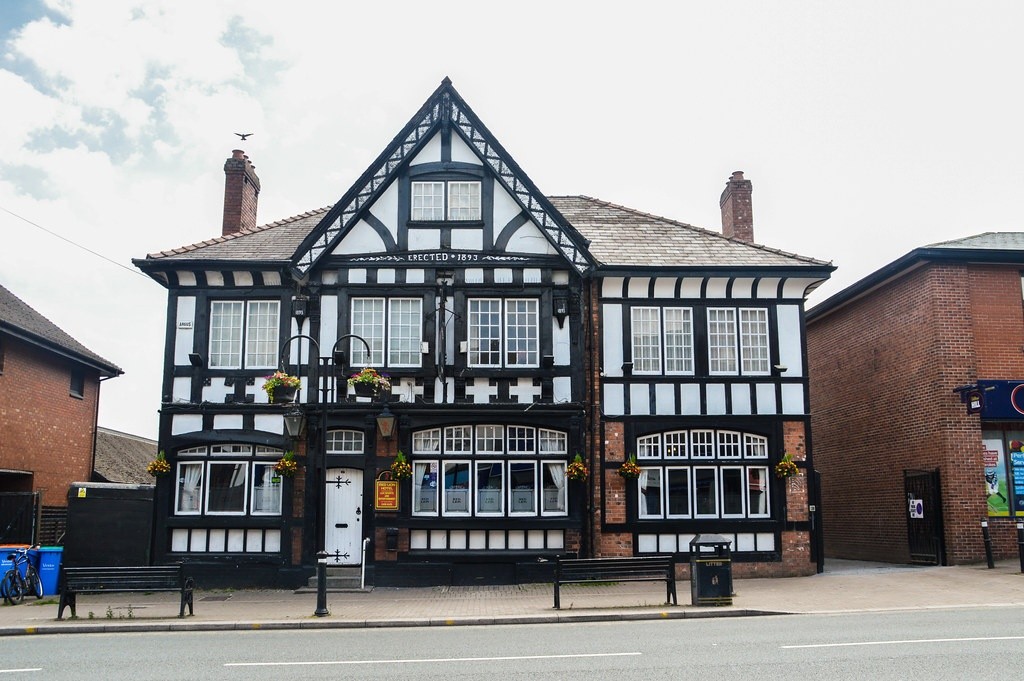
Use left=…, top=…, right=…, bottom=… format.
left=543, top=354, right=555, bottom=366
left=377, top=396, right=396, bottom=439
left=188, top=354, right=204, bottom=372
left=620, top=362, right=634, bottom=371
left=334, top=349, right=345, bottom=365
left=286, top=296, right=309, bottom=334
left=551, top=296, right=572, bottom=329
left=283, top=404, right=305, bottom=442
left=773, top=365, right=788, bottom=374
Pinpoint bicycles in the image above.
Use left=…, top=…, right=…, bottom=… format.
left=3, top=542, right=45, bottom=605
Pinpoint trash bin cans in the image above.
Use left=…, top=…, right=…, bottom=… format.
left=37, top=545, right=64, bottom=596
left=0, top=544, right=37, bottom=598
left=689, top=531, right=734, bottom=607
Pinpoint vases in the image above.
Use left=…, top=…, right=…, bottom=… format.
left=620, top=472, right=639, bottom=478
left=569, top=475, right=588, bottom=482
left=354, top=383, right=381, bottom=396
left=394, top=472, right=410, bottom=481
left=268, top=386, right=296, bottom=402
left=781, top=472, right=792, bottom=480
left=276, top=468, right=295, bottom=476
left=154, top=468, right=168, bottom=477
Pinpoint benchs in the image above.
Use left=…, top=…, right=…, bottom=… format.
left=552, top=555, right=677, bottom=610
left=57, top=562, right=195, bottom=621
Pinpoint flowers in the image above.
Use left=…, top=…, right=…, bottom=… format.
left=275, top=452, right=299, bottom=474
left=618, top=452, right=643, bottom=477
left=774, top=454, right=800, bottom=478
left=264, top=371, right=302, bottom=403
left=148, top=451, right=169, bottom=477
left=348, top=366, right=392, bottom=396
left=391, top=449, right=411, bottom=481
left=565, top=454, right=590, bottom=482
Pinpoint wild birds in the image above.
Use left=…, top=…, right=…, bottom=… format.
left=233, top=132, right=254, bottom=141
